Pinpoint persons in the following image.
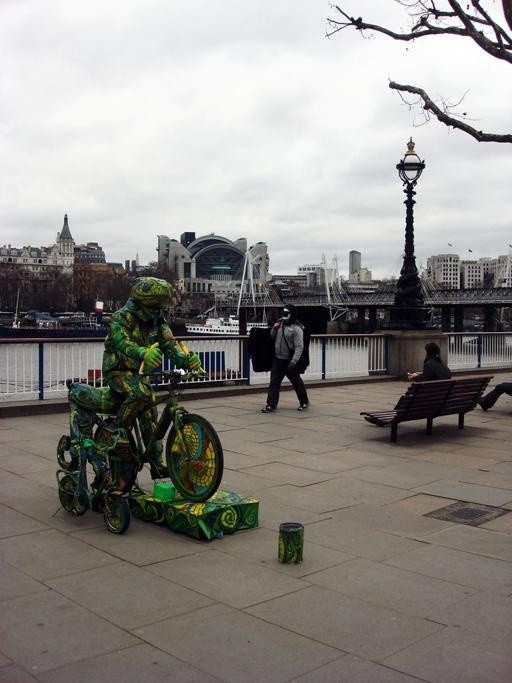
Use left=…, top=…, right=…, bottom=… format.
left=261, top=304, right=309, bottom=412
left=101, top=276, right=202, bottom=479
left=376, top=342, right=451, bottom=430
left=477, top=382, right=512, bottom=411
left=67, top=381, right=127, bottom=516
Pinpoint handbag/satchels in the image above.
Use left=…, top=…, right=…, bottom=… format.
left=288, top=347, right=303, bottom=360
left=250, top=327, right=310, bottom=372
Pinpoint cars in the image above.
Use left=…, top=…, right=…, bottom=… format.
left=61, top=311, right=86, bottom=318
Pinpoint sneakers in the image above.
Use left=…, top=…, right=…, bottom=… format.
left=262, top=403, right=275, bottom=414
left=296, top=403, right=310, bottom=411
left=477, top=398, right=488, bottom=411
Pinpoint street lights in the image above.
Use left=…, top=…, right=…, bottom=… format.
left=383, top=136, right=443, bottom=331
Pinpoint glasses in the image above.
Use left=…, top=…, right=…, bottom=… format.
left=280, top=311, right=290, bottom=317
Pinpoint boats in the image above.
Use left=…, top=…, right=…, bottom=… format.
left=0, top=314, right=108, bottom=338
left=185, top=239, right=305, bottom=335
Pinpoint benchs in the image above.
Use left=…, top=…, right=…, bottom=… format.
left=359, top=371, right=494, bottom=444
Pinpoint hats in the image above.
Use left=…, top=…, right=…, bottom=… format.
left=284, top=304, right=297, bottom=322
left=425, top=343, right=440, bottom=356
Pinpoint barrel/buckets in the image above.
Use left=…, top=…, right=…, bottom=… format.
left=278, top=522, right=304, bottom=565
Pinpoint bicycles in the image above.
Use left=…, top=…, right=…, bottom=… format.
left=56, top=340, right=224, bottom=534
